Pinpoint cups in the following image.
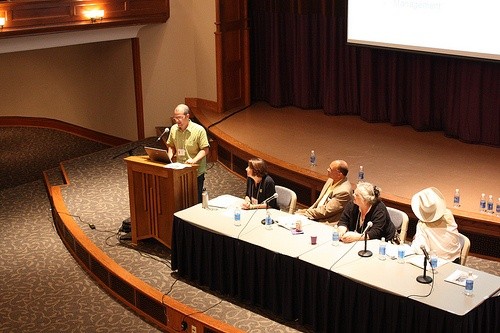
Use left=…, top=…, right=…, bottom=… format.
left=311, top=236, right=316, bottom=244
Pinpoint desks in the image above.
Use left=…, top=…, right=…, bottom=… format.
left=171, top=194, right=500, bottom=333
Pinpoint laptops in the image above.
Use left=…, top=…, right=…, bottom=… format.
left=143, top=147, right=186, bottom=164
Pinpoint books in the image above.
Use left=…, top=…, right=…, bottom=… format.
left=408, top=254, right=448, bottom=272
left=445, top=270, right=477, bottom=287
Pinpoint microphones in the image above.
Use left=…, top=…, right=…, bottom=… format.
left=363, top=221, right=373, bottom=235
left=262, top=193, right=278, bottom=203
left=157, top=127, right=170, bottom=141
left=420, top=244, right=430, bottom=262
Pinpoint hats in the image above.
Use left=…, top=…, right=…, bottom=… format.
left=411, top=186, right=446, bottom=222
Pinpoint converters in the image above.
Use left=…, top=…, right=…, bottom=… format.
left=90, top=223, right=96, bottom=229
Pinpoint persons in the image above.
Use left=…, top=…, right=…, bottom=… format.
left=293, top=159, right=353, bottom=225
left=409, top=186, right=461, bottom=265
left=336, top=182, right=400, bottom=246
left=167, top=104, right=210, bottom=203
left=241, top=158, right=280, bottom=210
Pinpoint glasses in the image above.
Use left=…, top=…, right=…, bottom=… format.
left=173, top=115, right=183, bottom=119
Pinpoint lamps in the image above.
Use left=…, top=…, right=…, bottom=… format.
left=0, top=18, right=5, bottom=29
left=89, top=10, right=104, bottom=24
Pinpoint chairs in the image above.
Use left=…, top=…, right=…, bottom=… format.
left=274, top=185, right=297, bottom=214
left=385, top=206, right=409, bottom=244
left=458, top=232, right=471, bottom=266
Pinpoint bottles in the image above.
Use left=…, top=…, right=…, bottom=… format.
left=233, top=207, right=241, bottom=226
left=378, top=237, right=386, bottom=260
left=464, top=271, right=474, bottom=296
left=397, top=241, right=405, bottom=263
left=265, top=213, right=272, bottom=230
left=453, top=188, right=460, bottom=209
left=202, top=187, right=208, bottom=208
left=480, top=193, right=487, bottom=213
left=332, top=224, right=340, bottom=245
left=487, top=195, right=494, bottom=215
left=430, top=250, right=438, bottom=273
left=496, top=198, right=500, bottom=217
left=358, top=165, right=365, bottom=181
left=296, top=219, right=302, bottom=232
left=310, top=150, right=317, bottom=167
